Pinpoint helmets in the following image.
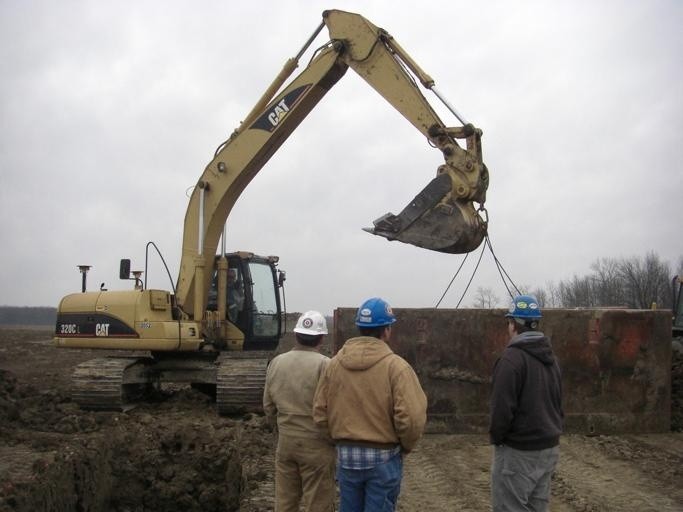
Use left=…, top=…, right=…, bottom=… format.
left=504, top=295, right=544, bottom=318
left=354, top=298, right=397, bottom=327
left=292, top=311, right=329, bottom=336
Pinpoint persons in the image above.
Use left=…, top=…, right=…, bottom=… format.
left=227, top=269, right=237, bottom=289
left=263, top=310, right=337, bottom=512
left=313, top=297, right=429, bottom=512
left=487, top=293, right=565, bottom=512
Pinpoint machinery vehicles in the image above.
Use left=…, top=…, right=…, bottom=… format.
left=52, top=9, right=490, bottom=420
